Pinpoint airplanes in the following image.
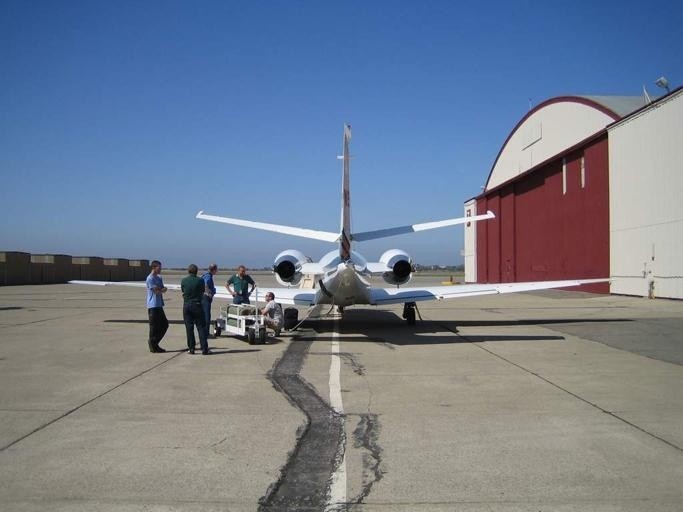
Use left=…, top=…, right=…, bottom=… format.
left=67, top=123, right=617, bottom=332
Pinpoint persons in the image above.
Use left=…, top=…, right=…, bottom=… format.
left=144, top=259, right=169, bottom=353
left=179, top=263, right=213, bottom=356
left=254, top=292, right=284, bottom=337
left=200, top=263, right=218, bottom=340
left=224, top=265, right=257, bottom=305
